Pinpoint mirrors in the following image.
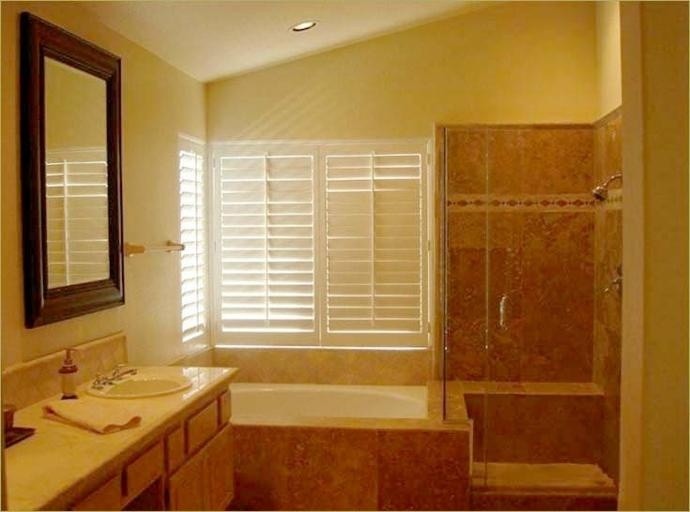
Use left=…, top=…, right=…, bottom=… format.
left=19, top=9, right=129, bottom=329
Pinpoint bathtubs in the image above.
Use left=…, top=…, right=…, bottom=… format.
left=227, top=380, right=429, bottom=420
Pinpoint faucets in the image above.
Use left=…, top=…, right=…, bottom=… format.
left=92, top=368, right=139, bottom=388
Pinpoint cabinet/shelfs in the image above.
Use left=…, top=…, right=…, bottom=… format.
left=167, top=419, right=237, bottom=511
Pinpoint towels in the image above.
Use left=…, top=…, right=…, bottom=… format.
left=44, top=396, right=145, bottom=436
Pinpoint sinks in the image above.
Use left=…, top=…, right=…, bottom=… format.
left=82, top=375, right=193, bottom=399
